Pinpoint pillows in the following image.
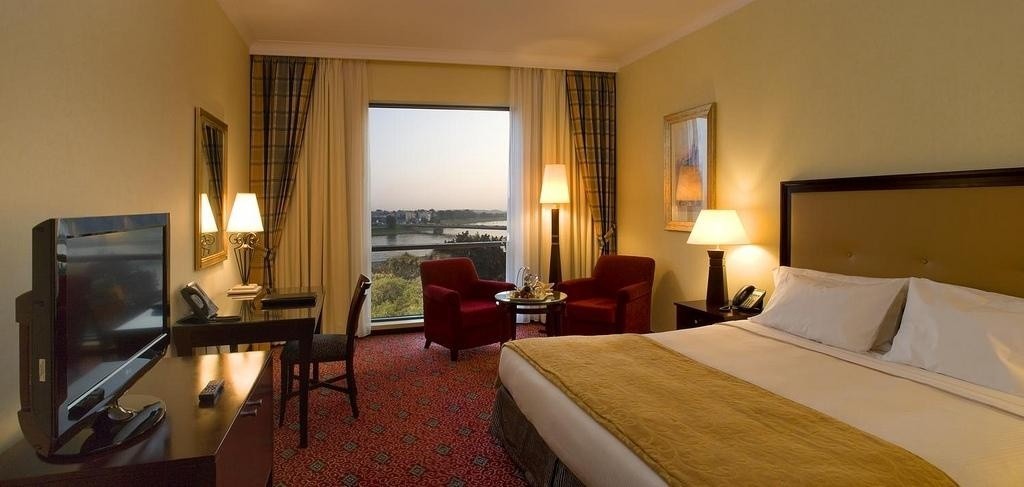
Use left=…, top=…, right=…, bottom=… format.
left=748, top=270, right=907, bottom=354
left=771, top=266, right=910, bottom=352
left=882, top=276, right=1024, bottom=400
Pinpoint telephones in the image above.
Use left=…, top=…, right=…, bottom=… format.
left=180, top=282, right=219, bottom=320
left=731, top=284, right=766, bottom=310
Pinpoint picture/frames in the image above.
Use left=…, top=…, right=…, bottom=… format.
left=661, top=102, right=718, bottom=234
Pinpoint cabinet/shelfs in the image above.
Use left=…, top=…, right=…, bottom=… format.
left=58, top=356, right=153, bottom=436
left=0, top=350, right=274, bottom=487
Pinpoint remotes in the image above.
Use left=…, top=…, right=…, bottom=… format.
left=198, top=379, right=224, bottom=399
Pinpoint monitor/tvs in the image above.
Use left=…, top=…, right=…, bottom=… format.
left=16, top=212, right=170, bottom=461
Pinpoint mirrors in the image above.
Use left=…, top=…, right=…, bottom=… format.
left=194, top=106, right=229, bottom=272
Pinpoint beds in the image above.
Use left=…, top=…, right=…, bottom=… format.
left=488, top=167, right=1024, bottom=487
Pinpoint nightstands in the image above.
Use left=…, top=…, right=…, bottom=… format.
left=674, top=298, right=762, bottom=331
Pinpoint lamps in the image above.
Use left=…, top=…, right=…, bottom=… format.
left=225, top=191, right=265, bottom=296
left=200, top=192, right=218, bottom=258
left=676, top=164, right=705, bottom=223
left=686, top=209, right=751, bottom=307
left=538, top=164, right=571, bottom=334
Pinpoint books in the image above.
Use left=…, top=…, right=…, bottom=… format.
left=260, top=292, right=317, bottom=308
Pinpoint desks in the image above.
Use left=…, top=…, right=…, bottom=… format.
left=172, top=285, right=328, bottom=449
left=112, top=308, right=163, bottom=359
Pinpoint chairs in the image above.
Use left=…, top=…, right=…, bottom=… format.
left=545, top=255, right=656, bottom=337
left=419, top=256, right=517, bottom=361
left=278, top=272, right=373, bottom=428
